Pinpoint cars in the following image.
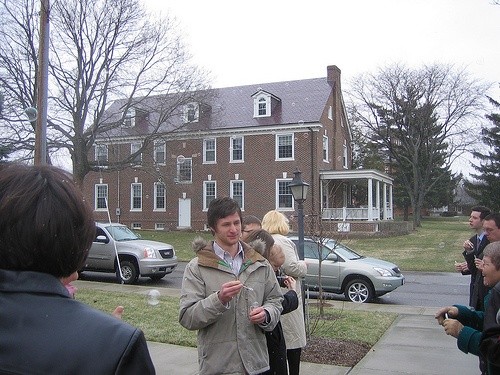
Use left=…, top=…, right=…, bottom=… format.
left=289, top=237, right=405, bottom=304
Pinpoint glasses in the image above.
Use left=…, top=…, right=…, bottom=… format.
left=242, top=230, right=253, bottom=233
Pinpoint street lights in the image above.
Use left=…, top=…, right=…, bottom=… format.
left=288, top=168, right=311, bottom=306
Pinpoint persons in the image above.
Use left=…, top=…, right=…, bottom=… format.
left=436, top=241, right=500, bottom=356
left=179, top=197, right=285, bottom=375
left=242, top=229, right=299, bottom=375
left=240, top=216, right=262, bottom=241
left=261, top=210, right=309, bottom=375
left=0, top=161, right=156, bottom=375
left=479, top=280, right=500, bottom=375
left=463, top=213, right=500, bottom=311
left=456, top=207, right=491, bottom=305
left=269, top=244, right=285, bottom=272
left=64, top=272, right=123, bottom=318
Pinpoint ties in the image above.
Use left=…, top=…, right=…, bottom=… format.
left=477, top=239, right=480, bottom=249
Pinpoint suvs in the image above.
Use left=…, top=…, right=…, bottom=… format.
left=84, top=223, right=178, bottom=285
